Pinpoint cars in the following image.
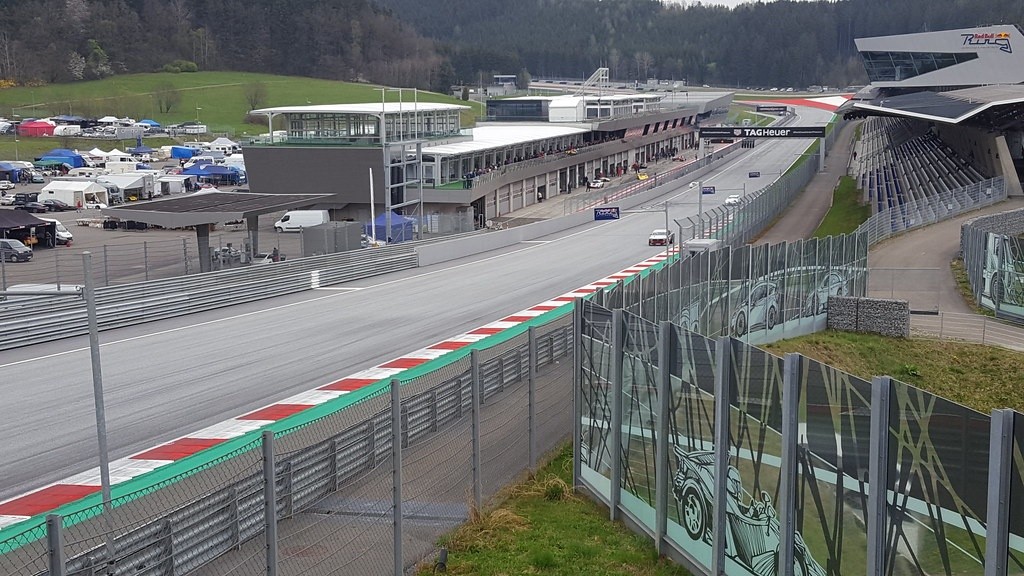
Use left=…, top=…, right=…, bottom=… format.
left=74, top=126, right=118, bottom=139
left=24, top=201, right=50, bottom=213
left=703, top=84, right=710, bottom=88
left=589, top=179, right=604, bottom=190
left=215, top=246, right=242, bottom=258
left=806, top=85, right=858, bottom=94
left=672, top=153, right=686, bottom=161
left=31, top=168, right=61, bottom=184
left=249, top=252, right=275, bottom=267
left=689, top=181, right=699, bottom=189
left=649, top=228, right=675, bottom=247
left=0, top=180, right=15, bottom=191
left=725, top=194, right=742, bottom=206
left=746, top=85, right=799, bottom=93
left=637, top=171, right=648, bottom=181
left=39, top=198, right=69, bottom=212
left=0, top=193, right=15, bottom=206
left=361, top=233, right=386, bottom=248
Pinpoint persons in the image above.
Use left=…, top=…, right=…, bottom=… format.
left=6, top=174, right=9, bottom=180
left=585, top=180, right=591, bottom=191
left=18, top=170, right=22, bottom=183
left=538, top=191, right=543, bottom=202
left=148, top=191, right=152, bottom=201
left=76, top=200, right=82, bottom=213
left=90, top=195, right=98, bottom=204
left=600, top=172, right=602, bottom=178
left=568, top=181, right=571, bottom=194
left=633, top=160, right=640, bottom=174
left=187, top=180, right=201, bottom=192
left=1, top=190, right=7, bottom=196
left=694, top=141, right=699, bottom=150
left=463, top=136, right=624, bottom=190
left=655, top=151, right=660, bottom=164
left=610, top=163, right=624, bottom=177
left=45, top=231, right=53, bottom=248
left=273, top=247, right=279, bottom=261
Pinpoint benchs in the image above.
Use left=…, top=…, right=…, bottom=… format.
left=858, top=117, right=999, bottom=234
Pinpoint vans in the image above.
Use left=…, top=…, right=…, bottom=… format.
left=132, top=123, right=152, bottom=132
left=16, top=191, right=40, bottom=205
left=184, top=155, right=215, bottom=169
left=0, top=238, right=34, bottom=262
left=19, top=217, right=73, bottom=246
left=273, top=210, right=331, bottom=233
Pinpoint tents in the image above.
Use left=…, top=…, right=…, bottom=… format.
left=0, top=208, right=56, bottom=250
left=130, top=146, right=158, bottom=163
left=362, top=209, right=413, bottom=243
left=37, top=181, right=108, bottom=210
left=0, top=163, right=17, bottom=183
left=33, top=160, right=63, bottom=177
left=182, top=162, right=237, bottom=187
